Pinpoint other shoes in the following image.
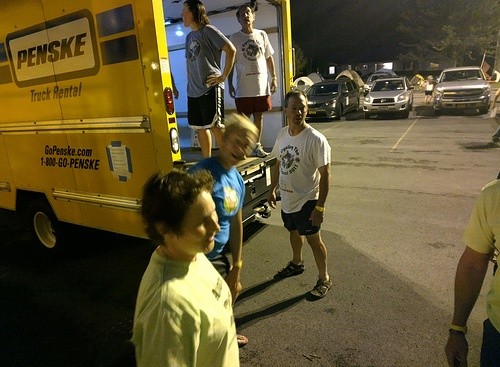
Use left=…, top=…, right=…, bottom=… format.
left=237, top=335, right=249, bottom=344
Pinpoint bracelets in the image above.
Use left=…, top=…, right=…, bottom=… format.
left=315, top=205, right=325, bottom=212
left=448, top=323, right=467, bottom=334
left=271, top=77, right=277, bottom=80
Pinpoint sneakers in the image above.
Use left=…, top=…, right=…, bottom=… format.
left=250, top=142, right=268, bottom=157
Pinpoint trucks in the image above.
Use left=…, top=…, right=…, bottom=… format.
left=0, top=0, right=297, bottom=272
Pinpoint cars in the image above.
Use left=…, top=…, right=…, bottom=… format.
left=429, top=66, right=491, bottom=115
left=362, top=77, right=414, bottom=119
left=364, top=73, right=389, bottom=97
left=305, top=79, right=360, bottom=121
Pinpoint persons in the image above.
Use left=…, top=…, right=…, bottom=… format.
left=422, top=75, right=433, bottom=104
left=185, top=113, right=259, bottom=344
left=267, top=89, right=333, bottom=298
left=226, top=5, right=277, bottom=158
left=181, top=0, right=237, bottom=159
left=491, top=88, right=500, bottom=142
left=444, top=179, right=500, bottom=367
left=133, top=168, right=240, bottom=367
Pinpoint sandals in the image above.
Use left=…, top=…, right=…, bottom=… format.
left=278, top=261, right=304, bottom=276
left=311, top=276, right=332, bottom=298
